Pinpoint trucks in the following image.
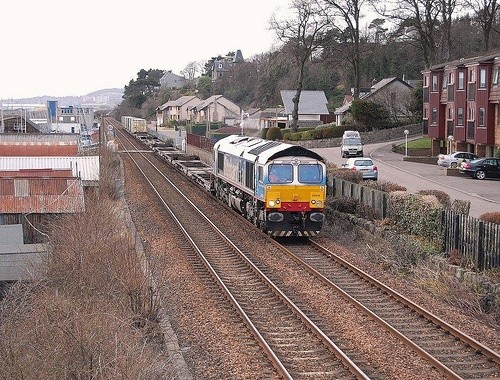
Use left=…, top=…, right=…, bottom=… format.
left=340, top=130, right=365, bottom=157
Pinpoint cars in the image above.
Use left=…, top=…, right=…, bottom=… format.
left=437, top=151, right=478, bottom=168
left=461, top=157, right=500, bottom=180
left=341, top=157, right=379, bottom=181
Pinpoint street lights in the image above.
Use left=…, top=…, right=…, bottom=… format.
left=448, top=135, right=455, bottom=154
left=404, top=129, right=409, bottom=156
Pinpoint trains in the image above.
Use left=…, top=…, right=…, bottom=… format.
left=213, top=136, right=328, bottom=239
